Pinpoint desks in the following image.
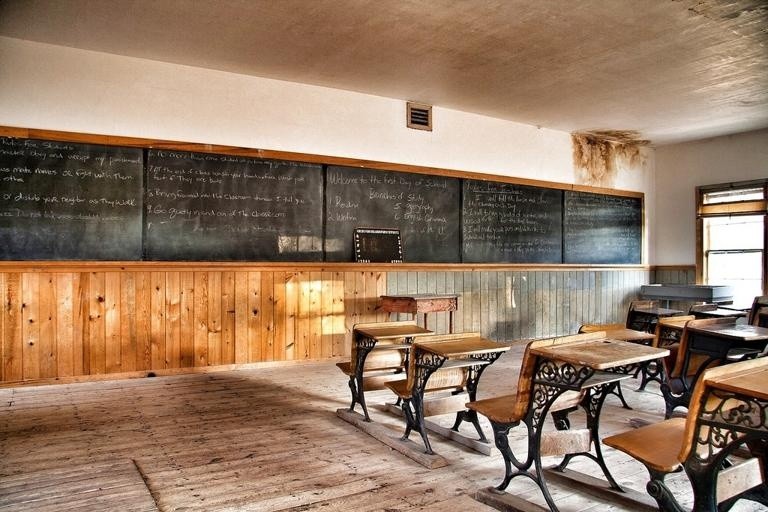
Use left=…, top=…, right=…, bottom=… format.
left=580, top=323, right=657, bottom=379
left=399, top=335, right=510, bottom=456
left=626, top=299, right=684, bottom=333
left=490, top=336, right=671, bottom=512
left=380, top=292, right=458, bottom=334
left=644, top=360, right=768, bottom=511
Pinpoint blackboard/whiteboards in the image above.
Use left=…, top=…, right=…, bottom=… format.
left=147, top=146, right=325, bottom=265
left=564, top=182, right=645, bottom=266
left=1, top=137, right=146, bottom=265
left=325, top=164, right=462, bottom=267
left=462, top=177, right=564, bottom=266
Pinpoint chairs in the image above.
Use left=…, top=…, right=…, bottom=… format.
left=661, top=316, right=768, bottom=420
left=465, top=329, right=670, bottom=512
left=384, top=332, right=510, bottom=454
left=601, top=357, right=768, bottom=512
